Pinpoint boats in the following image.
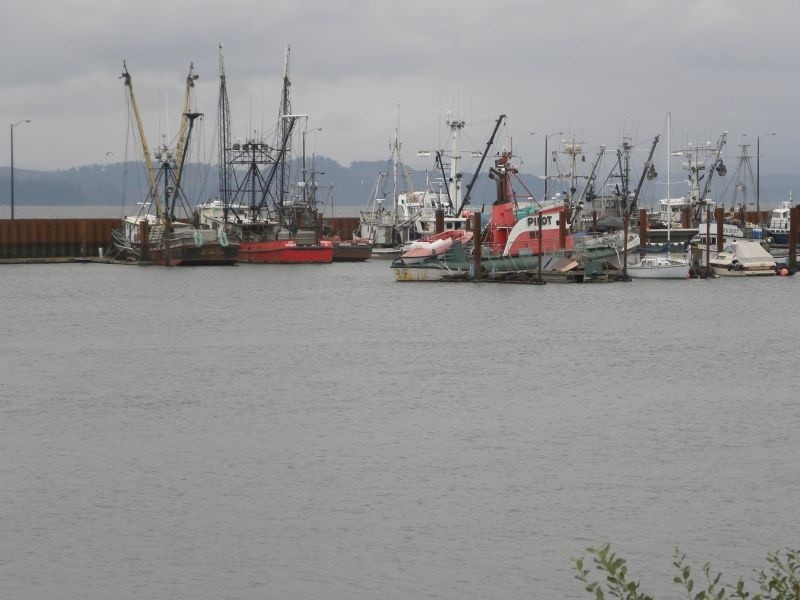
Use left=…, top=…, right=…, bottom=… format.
left=95, top=38, right=800, bottom=284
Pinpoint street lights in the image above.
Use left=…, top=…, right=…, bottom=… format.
left=530, top=130, right=564, bottom=200
left=302, top=127, right=323, bottom=202
left=742, top=132, right=777, bottom=224
left=10, top=120, right=30, bottom=219
